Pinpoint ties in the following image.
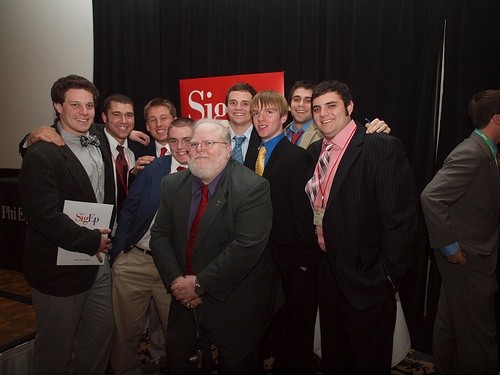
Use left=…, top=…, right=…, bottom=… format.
left=232, top=136, right=246, bottom=165
left=187, top=185, right=209, bottom=275
left=256, top=146, right=267, bottom=176
left=161, top=148, right=167, bottom=156
left=495, top=150, right=500, bottom=172
left=177, top=166, right=185, bottom=172
left=305, top=142, right=334, bottom=210
left=116, top=146, right=128, bottom=223
left=291, top=130, right=304, bottom=145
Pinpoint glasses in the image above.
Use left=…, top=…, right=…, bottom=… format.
left=190, top=140, right=227, bottom=150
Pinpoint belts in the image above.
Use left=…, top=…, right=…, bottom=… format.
left=134, top=245, right=153, bottom=256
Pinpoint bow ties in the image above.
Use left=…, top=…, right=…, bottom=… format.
left=80, top=135, right=100, bottom=147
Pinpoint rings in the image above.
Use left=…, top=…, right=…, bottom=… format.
left=187, top=303, right=189, bottom=307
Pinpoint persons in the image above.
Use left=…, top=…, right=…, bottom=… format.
left=107, top=118, right=196, bottom=375
left=420, top=89, right=500, bottom=375
left=284, top=80, right=390, bottom=150
left=127, top=98, right=177, bottom=191
left=150, top=119, right=272, bottom=374
left=225, top=83, right=261, bottom=171
left=17, top=74, right=151, bottom=375
left=297, top=80, right=418, bottom=375
left=250, top=90, right=320, bottom=375
left=19, top=94, right=145, bottom=237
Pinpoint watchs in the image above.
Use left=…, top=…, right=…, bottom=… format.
left=195, top=282, right=203, bottom=295
